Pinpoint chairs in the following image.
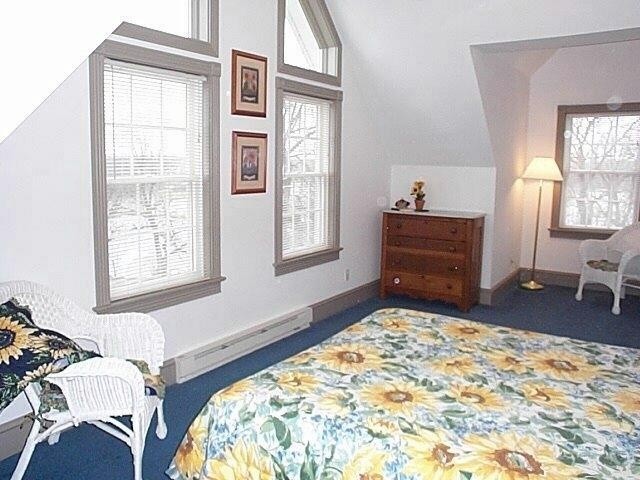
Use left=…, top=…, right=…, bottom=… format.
left=1, top=281, right=168, bottom=480
left=575, top=222, right=640, bottom=315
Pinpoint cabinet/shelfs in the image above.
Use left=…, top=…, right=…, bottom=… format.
left=380, top=209, right=487, bottom=313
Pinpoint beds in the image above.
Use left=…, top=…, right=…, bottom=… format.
left=165, top=307, right=640, bottom=480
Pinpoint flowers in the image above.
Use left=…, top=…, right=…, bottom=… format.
left=410, top=182, right=425, bottom=200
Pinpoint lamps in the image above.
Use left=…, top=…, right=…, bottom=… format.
left=518, top=158, right=564, bottom=290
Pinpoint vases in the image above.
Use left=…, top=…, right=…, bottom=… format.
left=414, top=200, right=425, bottom=210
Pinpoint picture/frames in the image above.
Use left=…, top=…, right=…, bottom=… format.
left=231, top=131, right=267, bottom=194
left=231, top=49, right=267, bottom=118
